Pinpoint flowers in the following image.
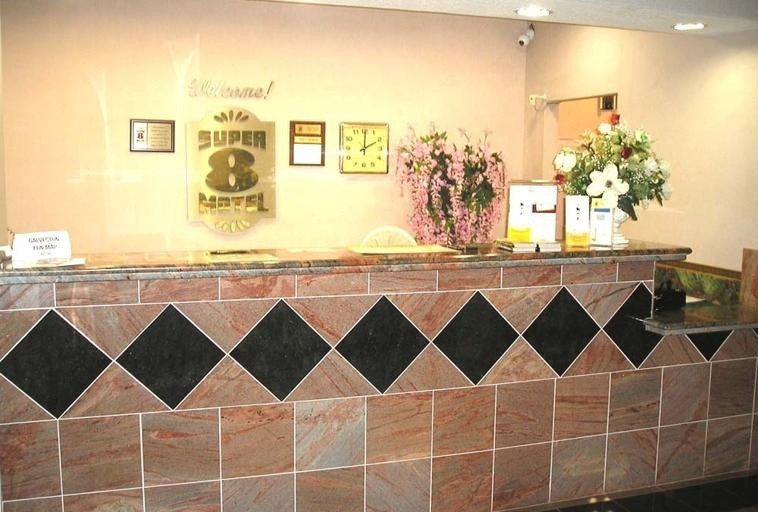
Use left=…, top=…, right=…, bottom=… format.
left=392, top=124, right=507, bottom=246
left=553, top=110, right=671, bottom=209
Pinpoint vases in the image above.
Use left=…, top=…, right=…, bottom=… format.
left=610, top=206, right=632, bottom=244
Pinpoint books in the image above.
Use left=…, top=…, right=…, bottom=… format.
left=497, top=236, right=560, bottom=252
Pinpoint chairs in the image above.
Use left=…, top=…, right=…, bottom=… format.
left=360, top=226, right=415, bottom=245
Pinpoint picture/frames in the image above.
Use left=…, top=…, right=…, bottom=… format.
left=129, top=118, right=175, bottom=153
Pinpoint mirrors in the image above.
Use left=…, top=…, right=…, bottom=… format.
left=288, top=121, right=325, bottom=166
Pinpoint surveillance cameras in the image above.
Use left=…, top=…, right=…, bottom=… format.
left=518, top=29, right=534, bottom=47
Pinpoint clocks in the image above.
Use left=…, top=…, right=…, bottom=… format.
left=339, top=122, right=388, bottom=174
